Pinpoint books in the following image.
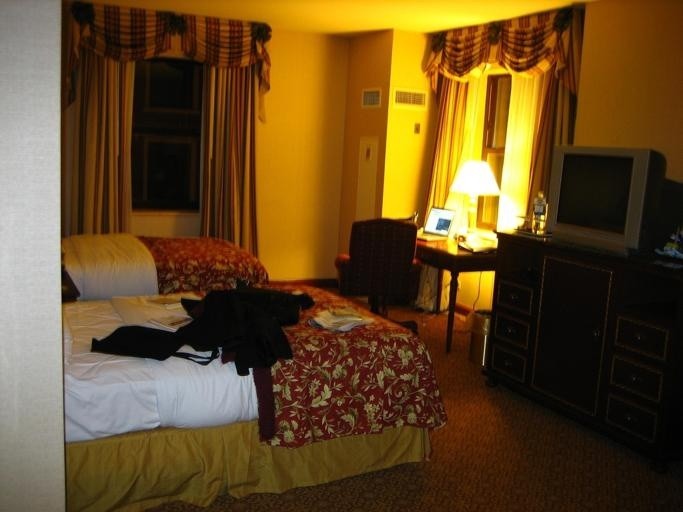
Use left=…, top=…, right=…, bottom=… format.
left=150, top=314, right=192, bottom=330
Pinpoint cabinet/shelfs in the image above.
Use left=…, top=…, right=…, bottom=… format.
left=528, top=241, right=618, bottom=420
left=485, top=235, right=543, bottom=385
left=598, top=254, right=681, bottom=445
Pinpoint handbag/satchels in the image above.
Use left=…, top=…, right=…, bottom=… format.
left=91, top=325, right=220, bottom=368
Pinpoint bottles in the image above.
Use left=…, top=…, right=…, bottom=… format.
left=528, top=187, right=551, bottom=235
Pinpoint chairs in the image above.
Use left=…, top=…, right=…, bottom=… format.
left=333, top=218, right=422, bottom=338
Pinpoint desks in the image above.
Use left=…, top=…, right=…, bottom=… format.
left=416, top=235, right=496, bottom=352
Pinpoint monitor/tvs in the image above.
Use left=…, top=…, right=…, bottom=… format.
left=546, top=144, right=683, bottom=257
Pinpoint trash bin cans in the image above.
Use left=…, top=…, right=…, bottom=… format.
left=469, top=310, right=492, bottom=368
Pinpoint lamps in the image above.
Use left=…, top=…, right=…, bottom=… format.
left=447, top=160, right=499, bottom=234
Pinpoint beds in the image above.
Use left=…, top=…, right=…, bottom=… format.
left=62, top=235, right=448, bottom=510
